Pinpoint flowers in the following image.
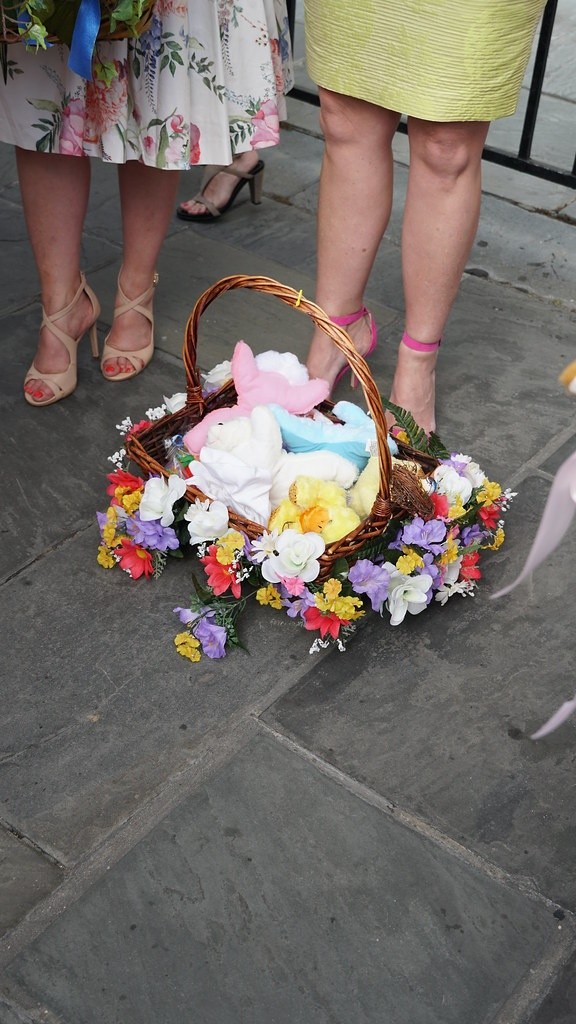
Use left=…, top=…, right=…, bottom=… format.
left=89, top=337, right=517, bottom=665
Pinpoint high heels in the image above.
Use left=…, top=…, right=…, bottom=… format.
left=177, top=147, right=265, bottom=219
left=304, top=306, right=441, bottom=446
left=25, top=265, right=160, bottom=406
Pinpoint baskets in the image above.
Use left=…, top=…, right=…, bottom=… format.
left=123, top=273, right=442, bottom=604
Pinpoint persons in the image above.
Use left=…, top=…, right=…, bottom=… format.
left=0, top=0, right=295, bottom=409
left=288, top=0, right=549, bottom=454
left=177, top=138, right=263, bottom=220
